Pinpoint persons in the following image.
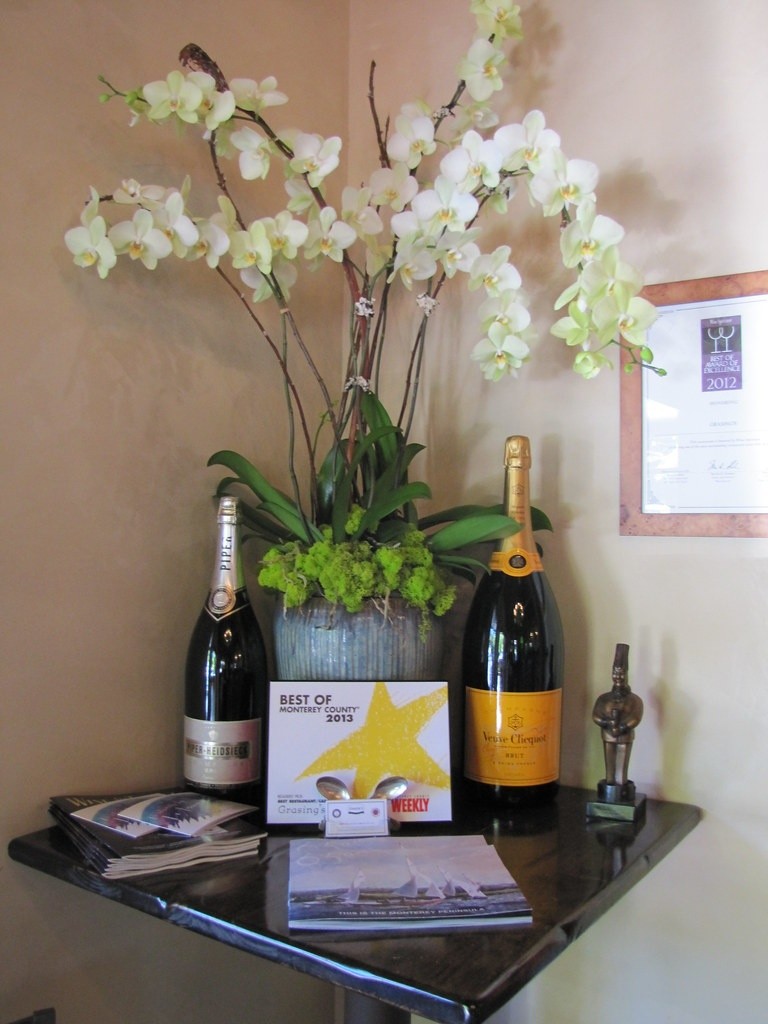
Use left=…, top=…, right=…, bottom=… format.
left=592, top=643, right=643, bottom=784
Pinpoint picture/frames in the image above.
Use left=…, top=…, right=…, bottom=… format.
left=620, top=270, right=768, bottom=538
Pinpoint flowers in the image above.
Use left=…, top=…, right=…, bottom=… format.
left=60, top=0, right=667, bottom=629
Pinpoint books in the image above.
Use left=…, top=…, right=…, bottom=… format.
left=50, top=784, right=269, bottom=881
left=287, top=836, right=534, bottom=931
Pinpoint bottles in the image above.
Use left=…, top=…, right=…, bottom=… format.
left=460, top=435, right=562, bottom=811
left=183, top=497, right=270, bottom=805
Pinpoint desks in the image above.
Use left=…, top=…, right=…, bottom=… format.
left=9, top=784, right=705, bottom=1024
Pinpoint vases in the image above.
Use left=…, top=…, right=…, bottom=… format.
left=272, top=592, right=444, bottom=679
